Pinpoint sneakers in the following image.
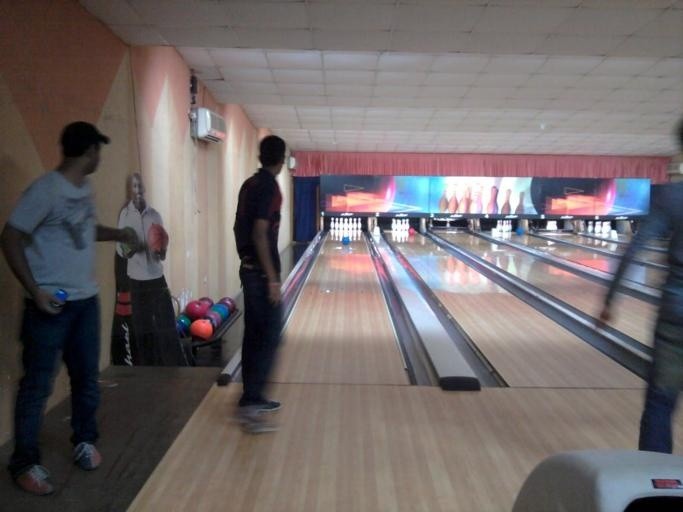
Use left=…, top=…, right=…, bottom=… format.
left=74, top=442, right=102, bottom=471
left=238, top=398, right=282, bottom=411
left=17, top=466, right=53, bottom=496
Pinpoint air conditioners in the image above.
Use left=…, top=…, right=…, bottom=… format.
left=190, top=106, right=226, bottom=144
left=287, top=156, right=297, bottom=169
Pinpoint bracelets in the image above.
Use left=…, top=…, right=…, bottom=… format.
left=267, top=282, right=281, bottom=288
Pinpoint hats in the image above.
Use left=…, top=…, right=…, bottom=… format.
left=61, top=121, right=110, bottom=146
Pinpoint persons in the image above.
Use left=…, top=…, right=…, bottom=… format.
left=0, top=122, right=140, bottom=496
left=115, top=173, right=189, bottom=366
left=232, top=135, right=286, bottom=411
left=598, top=118, right=683, bottom=458
